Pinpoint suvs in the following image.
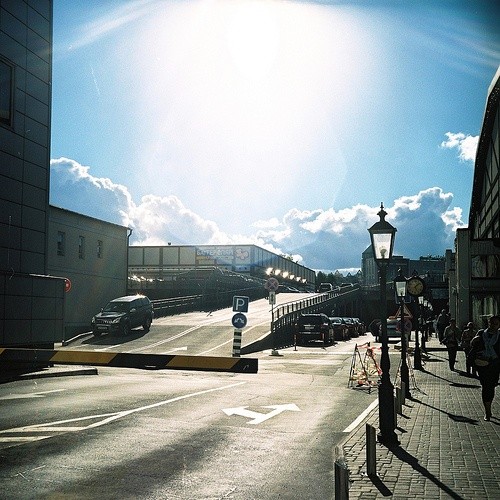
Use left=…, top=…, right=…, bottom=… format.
left=90, top=295, right=154, bottom=338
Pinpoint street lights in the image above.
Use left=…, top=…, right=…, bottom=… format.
left=417, top=293, right=434, bottom=353
left=392, top=266, right=413, bottom=398
left=367, top=199, right=400, bottom=445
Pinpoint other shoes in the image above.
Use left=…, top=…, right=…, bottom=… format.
left=484, top=407, right=492, bottom=421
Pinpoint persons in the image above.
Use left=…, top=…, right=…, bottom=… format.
left=461, top=321, right=477, bottom=376
left=442, top=319, right=462, bottom=372
left=466, top=314, right=500, bottom=421
left=436, top=323, right=448, bottom=344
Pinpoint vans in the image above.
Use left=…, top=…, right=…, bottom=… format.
left=292, top=312, right=335, bottom=346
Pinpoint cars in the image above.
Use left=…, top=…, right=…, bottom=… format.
left=318, top=283, right=332, bottom=293
left=274, top=284, right=301, bottom=295
left=327, top=316, right=367, bottom=341
left=378, top=315, right=399, bottom=342
left=340, top=282, right=353, bottom=287
